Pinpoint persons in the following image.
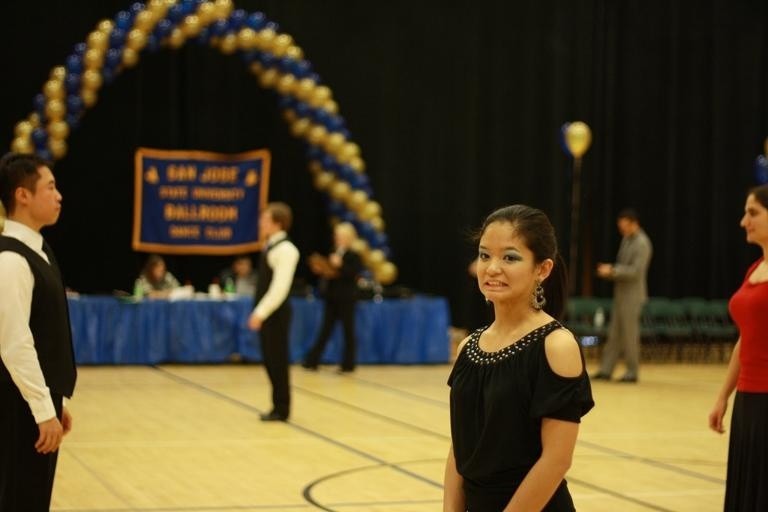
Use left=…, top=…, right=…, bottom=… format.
left=131, top=254, right=181, bottom=299
left=586, top=205, right=654, bottom=384
left=708, top=183, right=768, bottom=512
left=443, top=203, right=597, bottom=512
left=305, top=221, right=365, bottom=371
left=0, top=153, right=79, bottom=512
left=224, top=258, right=261, bottom=291
left=247, top=203, right=302, bottom=422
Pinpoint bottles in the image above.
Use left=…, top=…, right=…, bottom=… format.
left=142, top=277, right=154, bottom=302
left=225, top=276, right=238, bottom=300
left=208, top=276, right=222, bottom=300
left=132, top=276, right=145, bottom=301
left=592, top=306, right=606, bottom=333
left=181, top=279, right=193, bottom=302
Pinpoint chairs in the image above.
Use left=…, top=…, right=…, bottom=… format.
left=566, top=297, right=740, bottom=364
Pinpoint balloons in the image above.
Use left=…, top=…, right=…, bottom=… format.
left=557, top=120, right=592, bottom=159
left=1, top=1, right=401, bottom=291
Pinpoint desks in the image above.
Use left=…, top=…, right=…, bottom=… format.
left=68, top=293, right=450, bottom=367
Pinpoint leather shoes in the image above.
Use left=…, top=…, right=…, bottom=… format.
left=261, top=412, right=288, bottom=421
